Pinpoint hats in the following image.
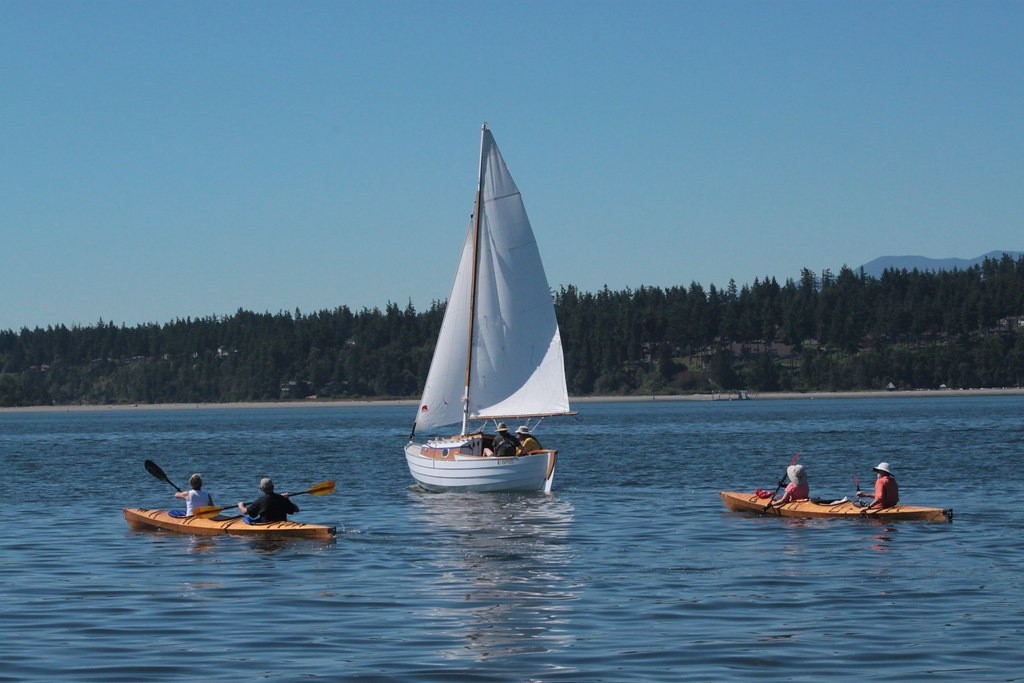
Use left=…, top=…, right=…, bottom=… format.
left=873, top=462, right=894, bottom=478
left=787, top=464, right=807, bottom=486
left=260, top=478, right=274, bottom=489
left=494, top=422, right=508, bottom=432
left=515, top=426, right=531, bottom=434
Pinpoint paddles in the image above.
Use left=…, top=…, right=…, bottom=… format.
left=195, top=479, right=335, bottom=520
left=144, top=457, right=185, bottom=493
left=759, top=452, right=799, bottom=516
left=852, top=472, right=868, bottom=516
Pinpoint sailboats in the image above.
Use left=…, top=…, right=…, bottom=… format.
left=403, top=121, right=579, bottom=495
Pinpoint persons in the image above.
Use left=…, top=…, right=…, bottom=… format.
left=238, top=478, right=299, bottom=524
left=856, top=462, right=899, bottom=513
left=771, top=464, right=809, bottom=507
left=175, top=474, right=214, bottom=517
left=515, top=426, right=542, bottom=455
left=483, top=423, right=525, bottom=458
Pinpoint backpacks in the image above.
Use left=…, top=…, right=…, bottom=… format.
left=496, top=439, right=515, bottom=456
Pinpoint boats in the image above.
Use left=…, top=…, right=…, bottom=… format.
left=719, top=490, right=954, bottom=523
left=122, top=508, right=336, bottom=541
